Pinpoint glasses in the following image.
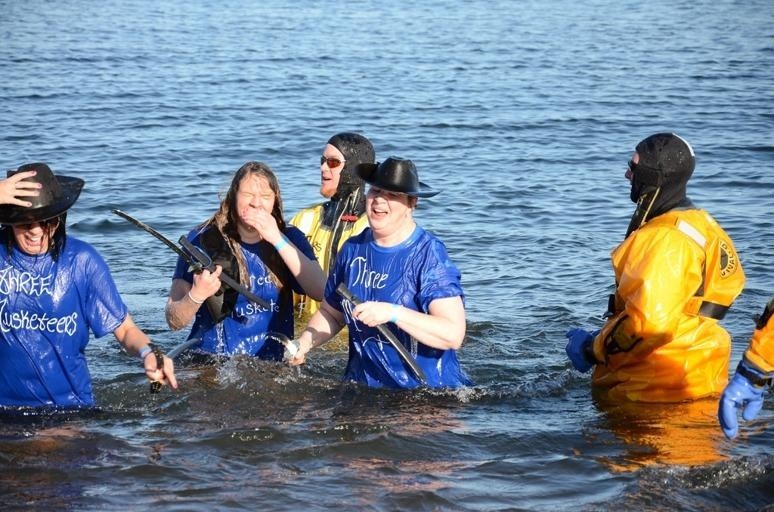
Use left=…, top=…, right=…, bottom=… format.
left=321, top=157, right=345, bottom=168
left=15, top=217, right=64, bottom=232
left=628, top=160, right=638, bottom=173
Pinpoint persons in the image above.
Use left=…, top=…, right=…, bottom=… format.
left=288, top=132, right=375, bottom=352
left=566, top=133, right=745, bottom=411
left=165, top=161, right=327, bottom=366
left=281, top=155, right=476, bottom=391
left=0, top=163, right=179, bottom=424
left=718, top=298, right=774, bottom=441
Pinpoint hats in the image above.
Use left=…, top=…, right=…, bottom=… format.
left=327, top=133, right=375, bottom=203
left=0, top=163, right=84, bottom=227
left=631, top=132, right=696, bottom=203
left=196, top=225, right=240, bottom=325
left=356, top=156, right=441, bottom=198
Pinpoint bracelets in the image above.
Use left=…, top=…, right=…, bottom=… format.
left=389, top=303, right=400, bottom=324
left=274, top=235, right=289, bottom=251
left=138, top=345, right=151, bottom=358
left=188, top=290, right=204, bottom=304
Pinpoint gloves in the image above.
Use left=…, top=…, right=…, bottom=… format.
left=718, top=360, right=774, bottom=440
left=565, top=328, right=600, bottom=374
